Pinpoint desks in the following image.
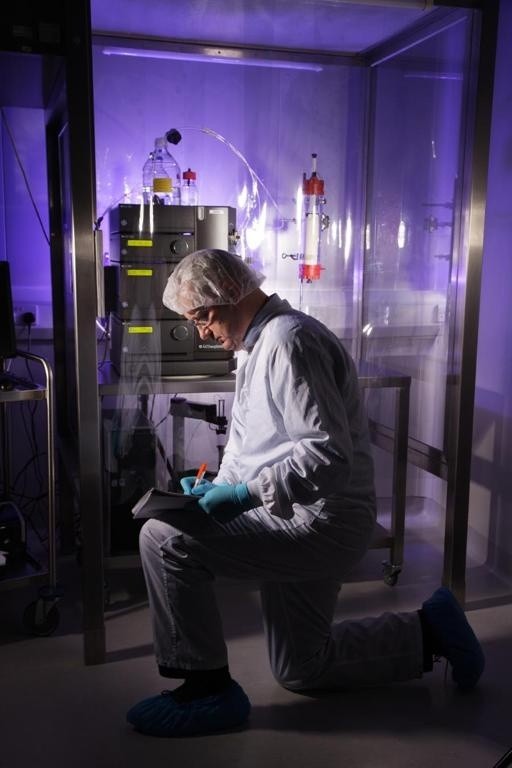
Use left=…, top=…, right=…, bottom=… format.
left=96, top=352, right=416, bottom=608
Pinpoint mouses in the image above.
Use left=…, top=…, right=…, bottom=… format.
left=0, top=378, right=15, bottom=391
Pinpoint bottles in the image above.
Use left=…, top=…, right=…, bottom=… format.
left=141, top=135, right=182, bottom=204
left=181, top=168, right=199, bottom=205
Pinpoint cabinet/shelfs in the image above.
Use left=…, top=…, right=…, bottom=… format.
left=0, top=349, right=62, bottom=631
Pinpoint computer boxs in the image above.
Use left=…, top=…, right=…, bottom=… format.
left=100, top=408, right=156, bottom=556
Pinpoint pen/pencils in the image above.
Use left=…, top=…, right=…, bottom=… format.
left=193, top=464, right=207, bottom=488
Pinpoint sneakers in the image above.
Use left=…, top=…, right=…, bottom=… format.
left=421, top=585, right=485, bottom=689
left=125, top=678, right=252, bottom=736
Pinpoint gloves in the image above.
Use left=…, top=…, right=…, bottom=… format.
left=197, top=482, right=258, bottom=524
left=180, top=475, right=219, bottom=497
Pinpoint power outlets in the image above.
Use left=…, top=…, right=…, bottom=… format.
left=14, top=302, right=40, bottom=327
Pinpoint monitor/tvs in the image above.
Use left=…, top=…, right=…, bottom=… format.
left=0, top=260, right=16, bottom=379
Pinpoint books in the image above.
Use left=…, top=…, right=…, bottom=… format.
left=130, top=487, right=205, bottom=520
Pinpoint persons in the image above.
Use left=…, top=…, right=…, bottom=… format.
left=124, top=247, right=489, bottom=737
